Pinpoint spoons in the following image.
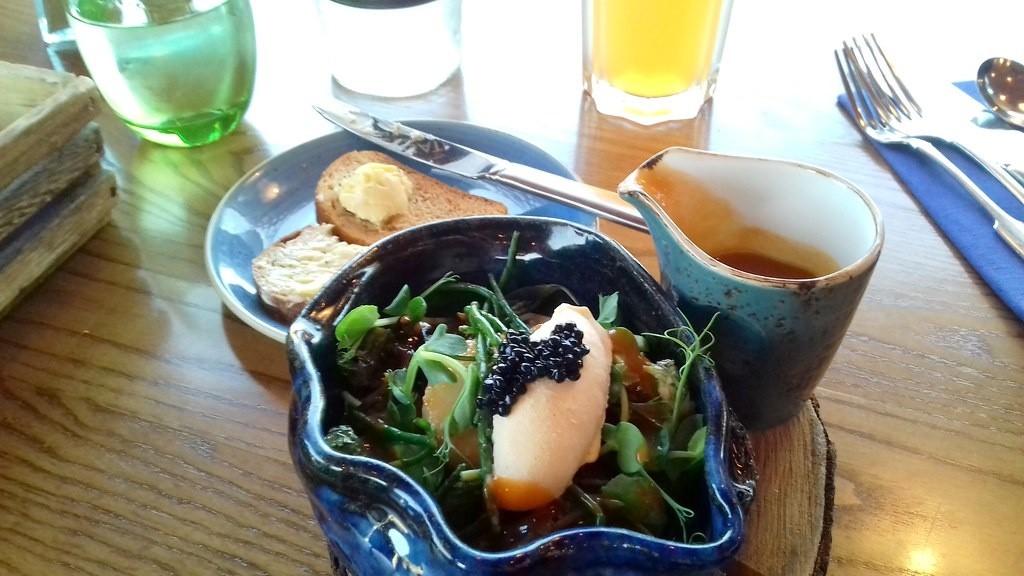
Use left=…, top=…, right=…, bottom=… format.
left=976, top=57, right=1024, bottom=127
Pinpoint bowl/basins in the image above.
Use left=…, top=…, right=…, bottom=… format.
left=289, top=215, right=744, bottom=576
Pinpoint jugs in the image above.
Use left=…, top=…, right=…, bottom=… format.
left=620, top=148, right=883, bottom=433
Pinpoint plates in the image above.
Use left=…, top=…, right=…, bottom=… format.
left=204, top=119, right=600, bottom=344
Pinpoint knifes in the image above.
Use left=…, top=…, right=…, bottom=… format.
left=311, top=100, right=649, bottom=233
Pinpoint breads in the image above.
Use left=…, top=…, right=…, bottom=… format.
left=251, top=150, right=509, bottom=322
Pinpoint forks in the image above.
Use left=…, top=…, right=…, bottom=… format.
left=835, top=31, right=1024, bottom=259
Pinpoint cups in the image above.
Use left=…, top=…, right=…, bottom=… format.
left=580, top=0, right=732, bottom=125
left=327, top=1, right=464, bottom=97
left=35, top=0, right=254, bottom=148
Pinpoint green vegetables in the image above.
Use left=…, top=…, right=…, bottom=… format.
left=334, top=233, right=720, bottom=546
